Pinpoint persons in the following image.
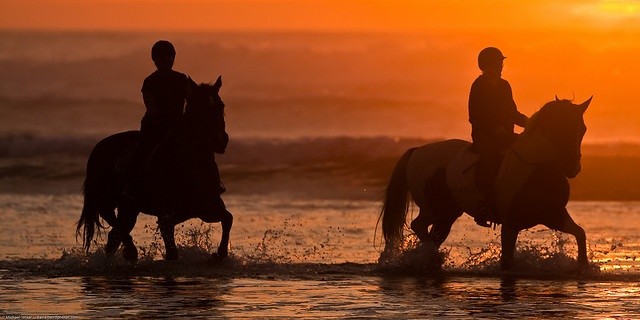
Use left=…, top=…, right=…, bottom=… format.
left=468, top=47, right=529, bottom=222
left=139, top=38, right=187, bottom=204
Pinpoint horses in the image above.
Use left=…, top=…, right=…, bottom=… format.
left=371, top=94, right=594, bottom=277
left=72, top=75, right=233, bottom=265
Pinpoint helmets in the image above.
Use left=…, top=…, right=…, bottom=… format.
left=151, top=39, right=176, bottom=61
left=478, top=48, right=507, bottom=67
